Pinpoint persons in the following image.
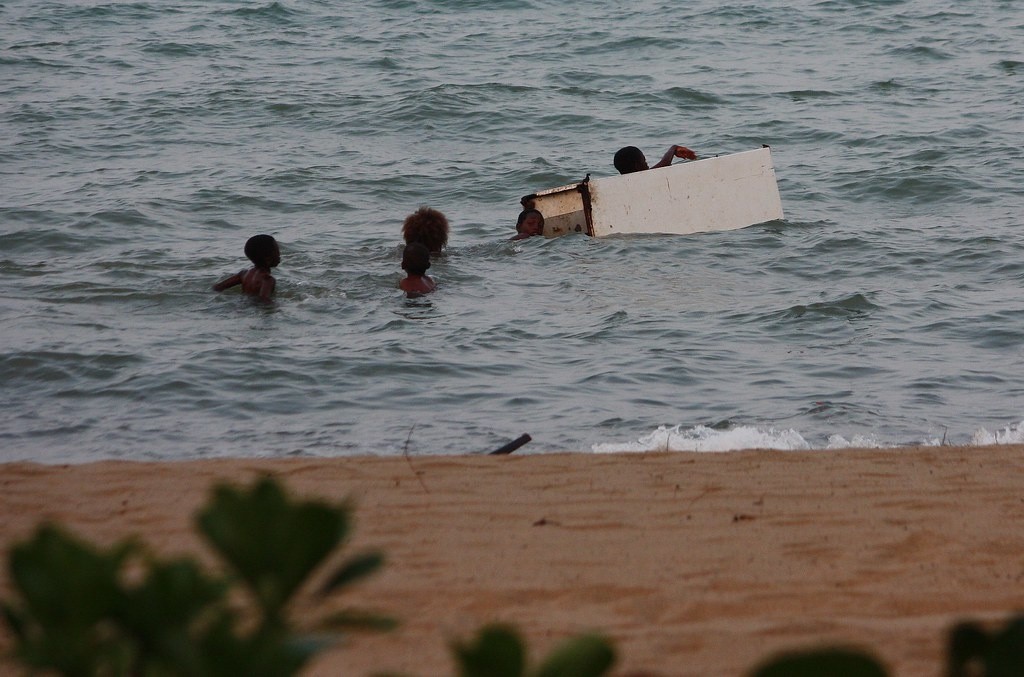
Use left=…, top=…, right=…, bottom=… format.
left=213, top=234, right=279, bottom=300
left=613, top=145, right=697, bottom=174
left=400, top=244, right=437, bottom=294
left=400, top=207, right=448, bottom=257
left=510, top=209, right=544, bottom=241
